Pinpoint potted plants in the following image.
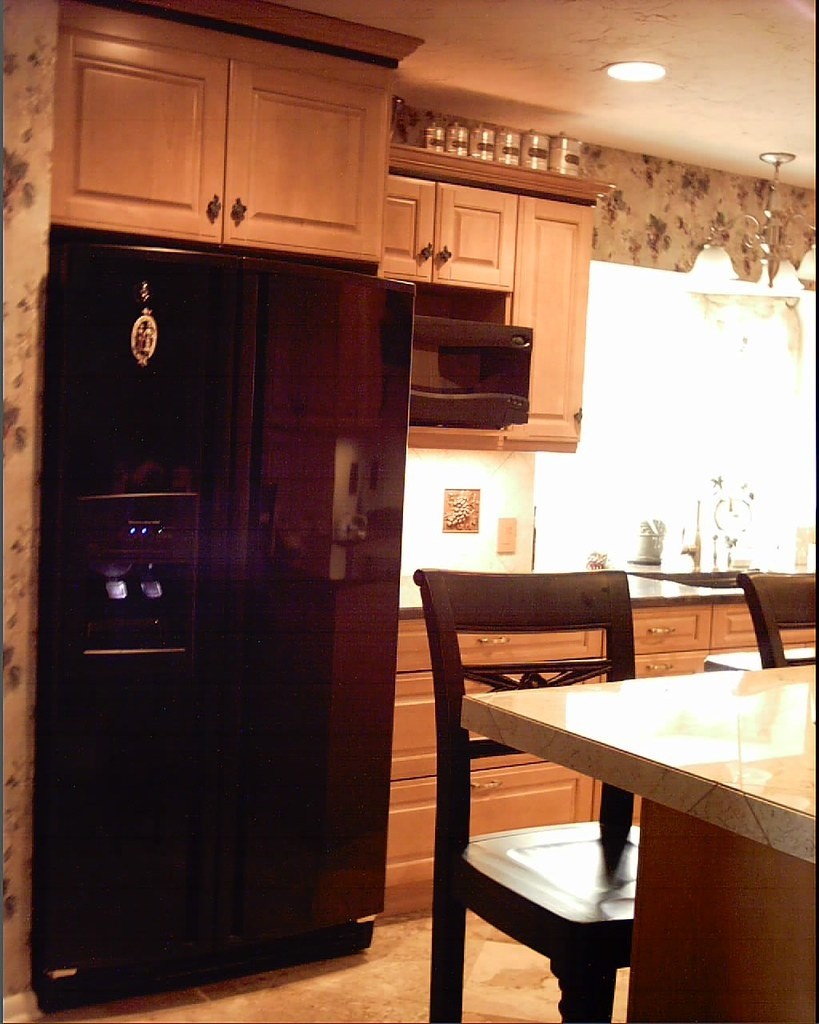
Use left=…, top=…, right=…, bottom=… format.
left=637, top=513, right=667, bottom=563
left=585, top=551, right=613, bottom=570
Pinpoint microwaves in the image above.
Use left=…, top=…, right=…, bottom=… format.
left=409, top=316, right=533, bottom=430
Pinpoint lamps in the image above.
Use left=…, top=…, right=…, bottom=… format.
left=682, top=153, right=815, bottom=294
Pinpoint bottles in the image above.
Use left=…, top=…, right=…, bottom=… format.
left=520, top=129, right=550, bottom=171
left=548, top=132, right=582, bottom=176
left=469, top=123, right=494, bottom=161
left=446, top=122, right=468, bottom=156
left=496, top=128, right=522, bottom=166
left=424, top=121, right=444, bottom=152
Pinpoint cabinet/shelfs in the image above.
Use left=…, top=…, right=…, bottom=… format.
left=369, top=142, right=520, bottom=295
left=504, top=164, right=616, bottom=454
left=377, top=590, right=817, bottom=941
left=52, top=0, right=425, bottom=260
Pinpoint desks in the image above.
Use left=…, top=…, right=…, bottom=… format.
left=462, top=659, right=816, bottom=1023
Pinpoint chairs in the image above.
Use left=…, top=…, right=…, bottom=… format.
left=416, top=570, right=642, bottom=1022
left=696, top=569, right=819, bottom=667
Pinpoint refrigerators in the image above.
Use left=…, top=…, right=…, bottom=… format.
left=30, top=246, right=412, bottom=1011
